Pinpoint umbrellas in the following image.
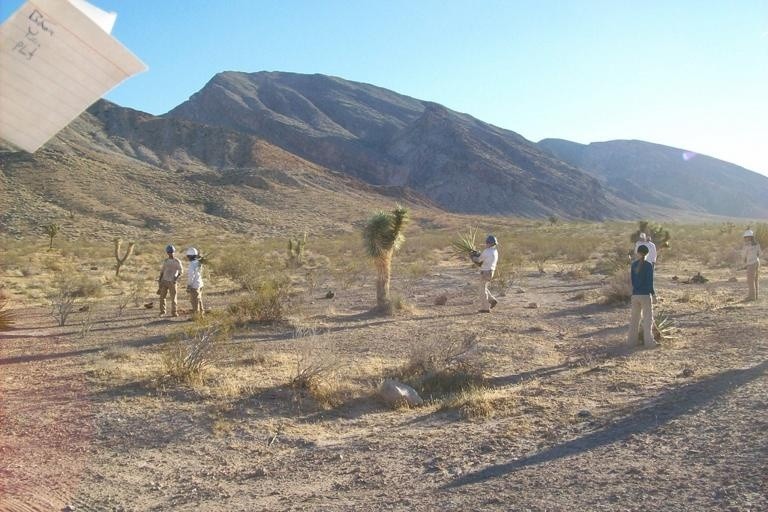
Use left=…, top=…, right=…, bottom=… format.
left=489, top=301, right=497, bottom=309
left=479, top=309, right=490, bottom=312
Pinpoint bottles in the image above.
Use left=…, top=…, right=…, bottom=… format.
left=640, top=232, right=646, bottom=239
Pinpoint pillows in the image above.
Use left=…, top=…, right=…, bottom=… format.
left=486, top=235, right=497, bottom=244
left=166, top=245, right=175, bottom=253
left=186, top=248, right=199, bottom=257
left=742, top=229, right=755, bottom=237
left=638, top=245, right=649, bottom=252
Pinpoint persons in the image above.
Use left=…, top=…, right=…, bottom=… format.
left=742, top=230, right=760, bottom=302
left=629, top=232, right=661, bottom=349
left=185, top=247, right=205, bottom=314
left=472, top=234, right=498, bottom=314
left=158, top=244, right=183, bottom=317
